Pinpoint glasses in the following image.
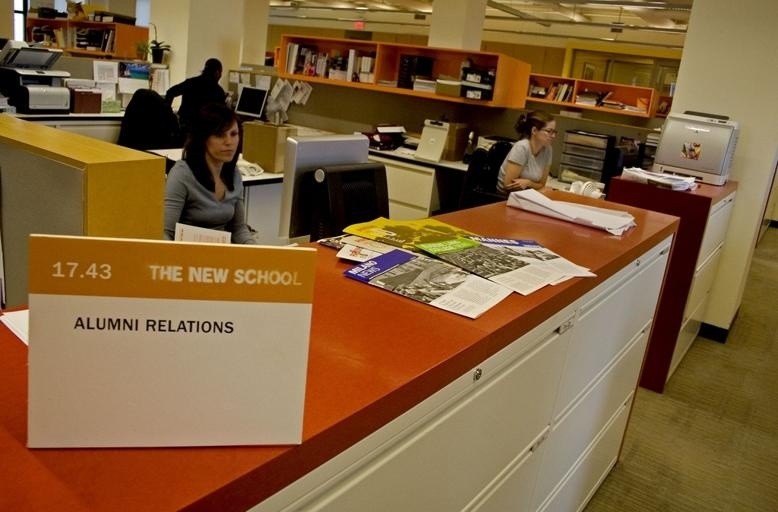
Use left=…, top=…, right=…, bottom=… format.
left=541, top=128, right=559, bottom=136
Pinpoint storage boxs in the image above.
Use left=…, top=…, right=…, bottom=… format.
left=435, top=73, right=464, bottom=96
left=69, top=88, right=101, bottom=114
left=414, top=118, right=470, bottom=164
left=558, top=130, right=616, bottom=195
left=242, top=121, right=297, bottom=173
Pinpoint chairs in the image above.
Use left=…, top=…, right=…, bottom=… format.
left=124, top=89, right=184, bottom=153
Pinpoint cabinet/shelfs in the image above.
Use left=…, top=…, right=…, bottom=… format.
left=277, top=34, right=532, bottom=109
left=44, top=121, right=123, bottom=145
left=525, top=73, right=661, bottom=119
left=26, top=18, right=150, bottom=60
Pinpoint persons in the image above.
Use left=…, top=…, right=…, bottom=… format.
left=163, top=105, right=257, bottom=245
left=495, top=110, right=556, bottom=195
left=166, top=58, right=225, bottom=130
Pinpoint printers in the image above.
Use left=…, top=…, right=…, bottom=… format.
left=651, top=110, right=740, bottom=186
left=0, top=47, right=70, bottom=114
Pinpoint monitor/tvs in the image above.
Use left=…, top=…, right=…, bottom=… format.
left=278, top=134, right=370, bottom=239
left=234, top=86, right=268, bottom=118
left=611, top=145, right=628, bottom=176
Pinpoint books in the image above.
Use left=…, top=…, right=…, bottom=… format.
left=52, top=26, right=114, bottom=52
left=527, top=79, right=648, bottom=113
left=285, top=43, right=374, bottom=83
left=316, top=216, right=597, bottom=319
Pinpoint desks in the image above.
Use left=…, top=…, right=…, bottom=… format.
left=146, top=145, right=285, bottom=239
left=284, top=122, right=606, bottom=218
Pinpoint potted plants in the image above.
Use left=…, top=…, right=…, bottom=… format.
left=137, top=22, right=170, bottom=63
